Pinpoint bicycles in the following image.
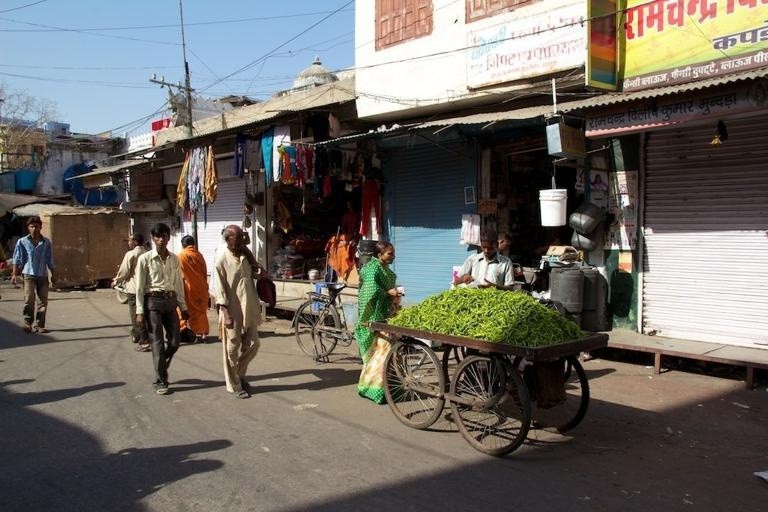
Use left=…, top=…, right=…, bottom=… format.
left=289, top=282, right=358, bottom=363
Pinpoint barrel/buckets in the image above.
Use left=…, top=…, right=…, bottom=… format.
left=568, top=202, right=606, bottom=249
left=539, top=189, right=567, bottom=227
left=549, top=267, right=606, bottom=331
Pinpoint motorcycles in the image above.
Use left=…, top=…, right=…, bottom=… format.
left=113, top=283, right=129, bottom=304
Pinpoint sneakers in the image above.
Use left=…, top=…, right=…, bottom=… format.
left=130, top=332, right=141, bottom=344
left=34, top=326, right=48, bottom=333
left=152, top=380, right=169, bottom=395
left=23, top=324, right=32, bottom=333
left=137, top=343, right=151, bottom=352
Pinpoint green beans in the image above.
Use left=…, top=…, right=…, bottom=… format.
left=384, top=287, right=588, bottom=347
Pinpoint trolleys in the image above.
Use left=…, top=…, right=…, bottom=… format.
left=361, top=320, right=609, bottom=457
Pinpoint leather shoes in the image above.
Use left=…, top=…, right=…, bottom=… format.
left=196, top=336, right=207, bottom=343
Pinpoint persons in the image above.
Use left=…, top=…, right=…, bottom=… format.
left=135, top=221, right=192, bottom=395
left=0, top=245, right=8, bottom=272
left=340, top=196, right=360, bottom=236
left=111, top=232, right=154, bottom=352
left=174, top=234, right=211, bottom=343
left=213, top=224, right=268, bottom=399
left=11, top=213, right=58, bottom=335
left=354, top=239, right=406, bottom=408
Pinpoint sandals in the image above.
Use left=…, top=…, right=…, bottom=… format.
left=227, top=387, right=249, bottom=399
left=239, top=377, right=252, bottom=391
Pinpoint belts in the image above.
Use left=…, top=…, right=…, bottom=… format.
left=145, top=290, right=176, bottom=299
